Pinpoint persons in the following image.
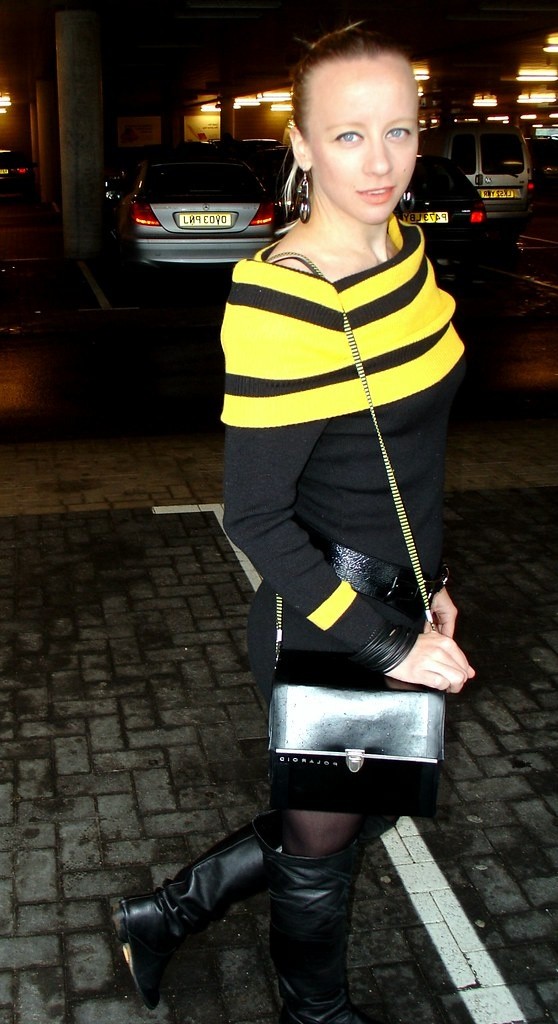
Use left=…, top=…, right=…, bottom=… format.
left=109, top=21, right=476, bottom=1024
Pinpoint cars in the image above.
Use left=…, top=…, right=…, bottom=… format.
left=0, top=148, right=39, bottom=198
left=102, top=138, right=293, bottom=214
left=523, top=138, right=558, bottom=194
left=114, top=157, right=276, bottom=283
left=400, top=152, right=489, bottom=282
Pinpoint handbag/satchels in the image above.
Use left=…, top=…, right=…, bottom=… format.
left=268, top=682, right=445, bottom=819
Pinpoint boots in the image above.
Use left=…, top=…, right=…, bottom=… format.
left=252, top=811, right=379, bottom=1024
left=111, top=813, right=288, bottom=1010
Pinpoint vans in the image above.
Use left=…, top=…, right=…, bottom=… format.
left=414, top=120, right=536, bottom=226
